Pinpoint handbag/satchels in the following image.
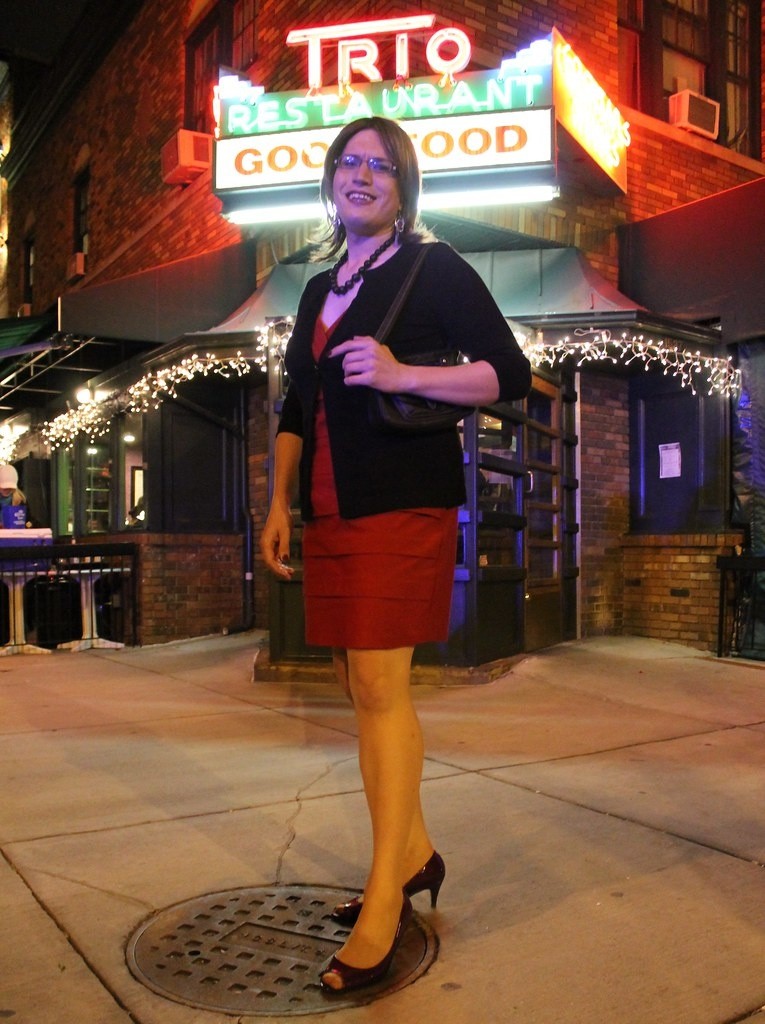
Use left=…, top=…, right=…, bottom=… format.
left=370, top=243, right=477, bottom=430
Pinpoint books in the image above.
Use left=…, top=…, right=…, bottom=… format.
left=2, top=505, right=25, bottom=528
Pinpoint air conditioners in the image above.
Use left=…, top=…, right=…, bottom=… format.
left=666, top=89, right=720, bottom=139
left=159, top=131, right=215, bottom=185
left=16, top=303, right=33, bottom=317
left=65, top=252, right=87, bottom=282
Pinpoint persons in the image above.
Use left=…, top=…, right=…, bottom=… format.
left=259, top=116, right=532, bottom=995
left=0, top=464, right=27, bottom=529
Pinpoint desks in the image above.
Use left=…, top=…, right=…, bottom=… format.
left=48, top=566, right=132, bottom=654
left=0, top=571, right=47, bottom=657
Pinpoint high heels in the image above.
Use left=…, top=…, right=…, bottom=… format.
left=318, top=888, right=413, bottom=994
left=331, top=850, right=446, bottom=923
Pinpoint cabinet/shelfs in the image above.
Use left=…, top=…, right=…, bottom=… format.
left=68, top=439, right=112, bottom=533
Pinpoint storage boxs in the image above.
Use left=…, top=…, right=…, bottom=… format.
left=0, top=528, right=56, bottom=571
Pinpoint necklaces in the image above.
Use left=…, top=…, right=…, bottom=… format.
left=329, top=235, right=396, bottom=295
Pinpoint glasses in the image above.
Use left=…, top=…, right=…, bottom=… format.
left=334, top=154, right=398, bottom=173
